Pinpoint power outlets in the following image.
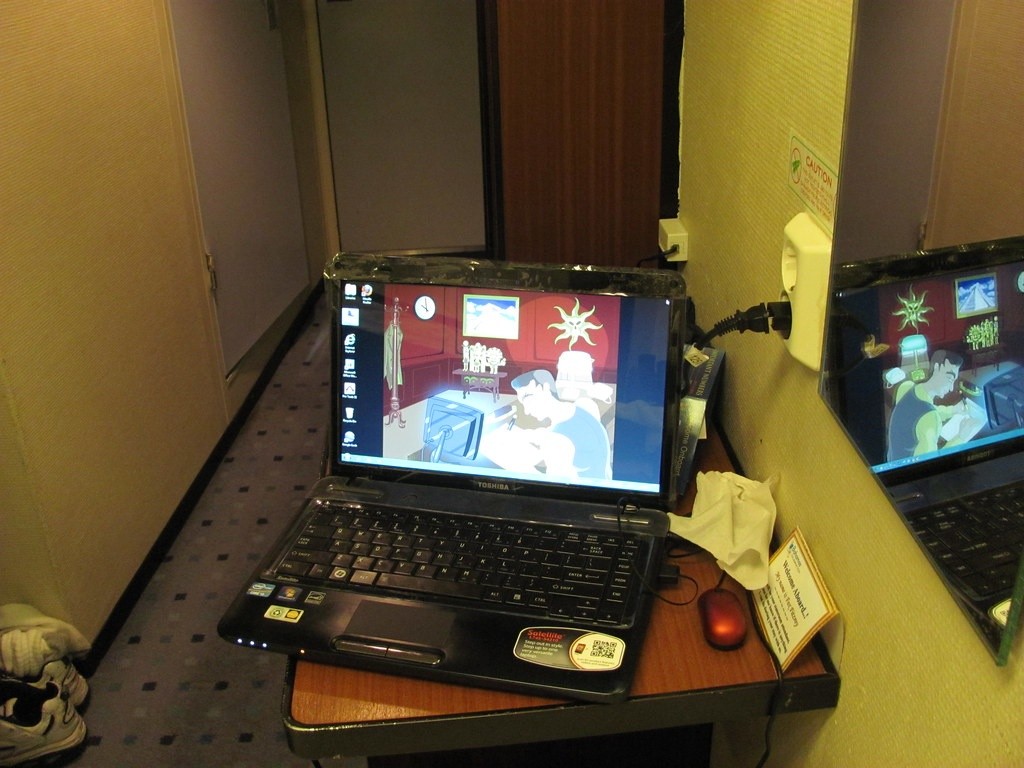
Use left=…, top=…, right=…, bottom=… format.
left=658, top=219, right=688, bottom=262
left=776, top=212, right=833, bottom=372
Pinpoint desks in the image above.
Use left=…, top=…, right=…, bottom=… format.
left=277, top=412, right=842, bottom=768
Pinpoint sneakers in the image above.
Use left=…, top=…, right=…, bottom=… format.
left=0, top=655, right=89, bottom=768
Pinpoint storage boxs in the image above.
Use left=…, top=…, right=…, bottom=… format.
left=670, top=342, right=726, bottom=495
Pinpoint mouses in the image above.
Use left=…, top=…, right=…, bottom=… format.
left=699, top=588, right=747, bottom=649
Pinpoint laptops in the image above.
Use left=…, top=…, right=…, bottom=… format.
left=218, top=253, right=686, bottom=707
left=821, top=235, right=1024, bottom=640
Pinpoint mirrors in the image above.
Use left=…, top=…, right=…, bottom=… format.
left=818, top=0, right=1024, bottom=666
